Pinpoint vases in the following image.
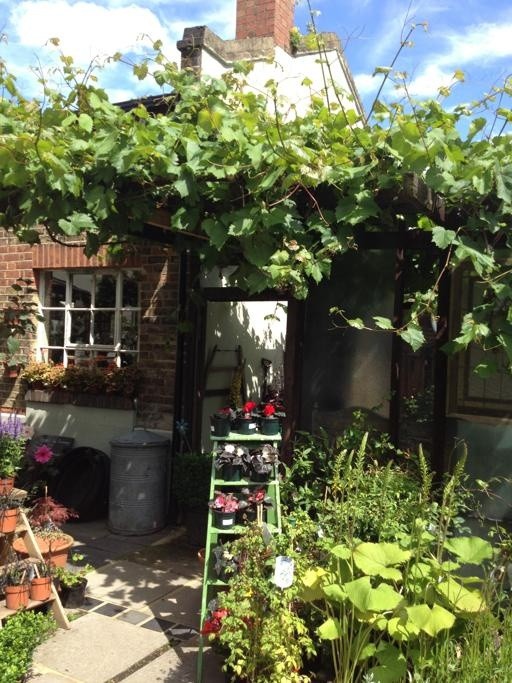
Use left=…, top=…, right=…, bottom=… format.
left=3, top=361, right=21, bottom=378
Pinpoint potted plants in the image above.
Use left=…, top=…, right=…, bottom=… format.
left=0, top=479, right=93, bottom=609
left=172, top=451, right=222, bottom=548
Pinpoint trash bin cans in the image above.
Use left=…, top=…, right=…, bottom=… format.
left=32, top=436, right=75, bottom=499
left=108, top=425, right=171, bottom=536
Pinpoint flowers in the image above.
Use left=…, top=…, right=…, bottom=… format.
left=0, top=408, right=53, bottom=479
left=18, top=354, right=144, bottom=397
left=198, top=400, right=287, bottom=634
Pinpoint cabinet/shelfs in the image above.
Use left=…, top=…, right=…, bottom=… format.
left=195, top=430, right=282, bottom=683
left=0, top=507, right=72, bottom=632
